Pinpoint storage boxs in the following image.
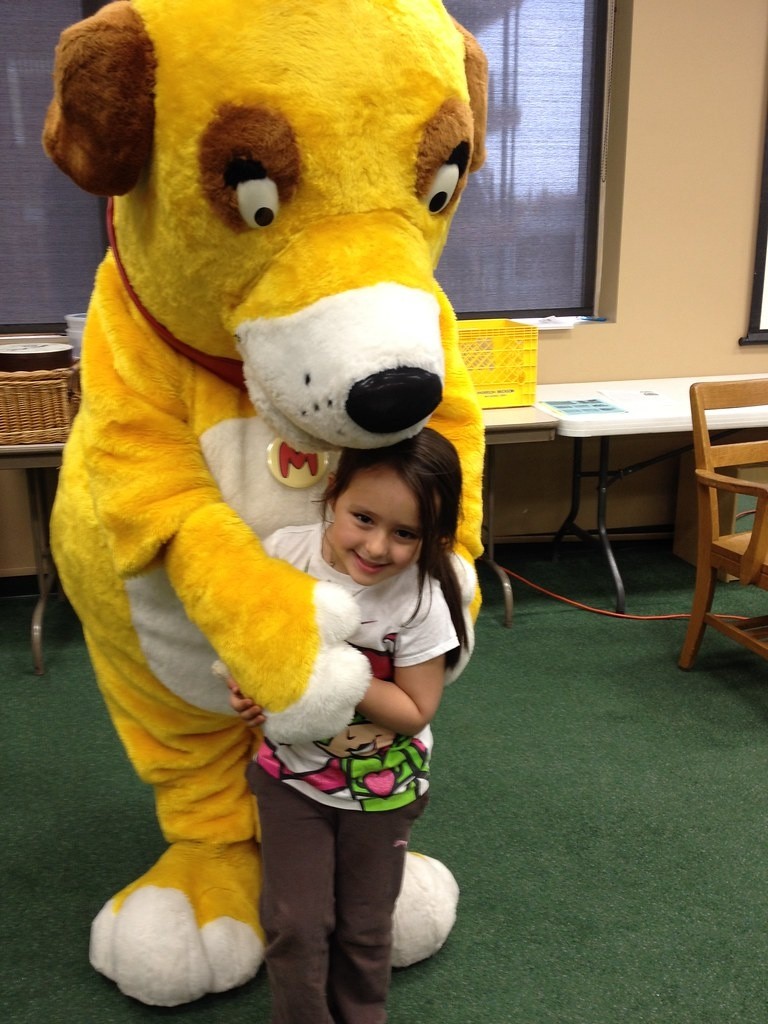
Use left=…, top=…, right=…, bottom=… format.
left=456, top=317, right=538, bottom=410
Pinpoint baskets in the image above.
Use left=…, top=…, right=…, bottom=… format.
left=455, top=317, right=539, bottom=409
left=0, top=361, right=81, bottom=444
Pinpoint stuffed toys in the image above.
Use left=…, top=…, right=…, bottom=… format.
left=43, top=1, right=486, bottom=1005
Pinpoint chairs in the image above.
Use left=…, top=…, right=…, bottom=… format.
left=679, top=379, right=768, bottom=677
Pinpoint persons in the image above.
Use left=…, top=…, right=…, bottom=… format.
left=226, top=426, right=470, bottom=1024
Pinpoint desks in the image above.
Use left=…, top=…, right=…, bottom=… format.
left=484, top=405, right=561, bottom=627
left=0, top=442, right=64, bottom=675
left=531, top=372, right=768, bottom=617
